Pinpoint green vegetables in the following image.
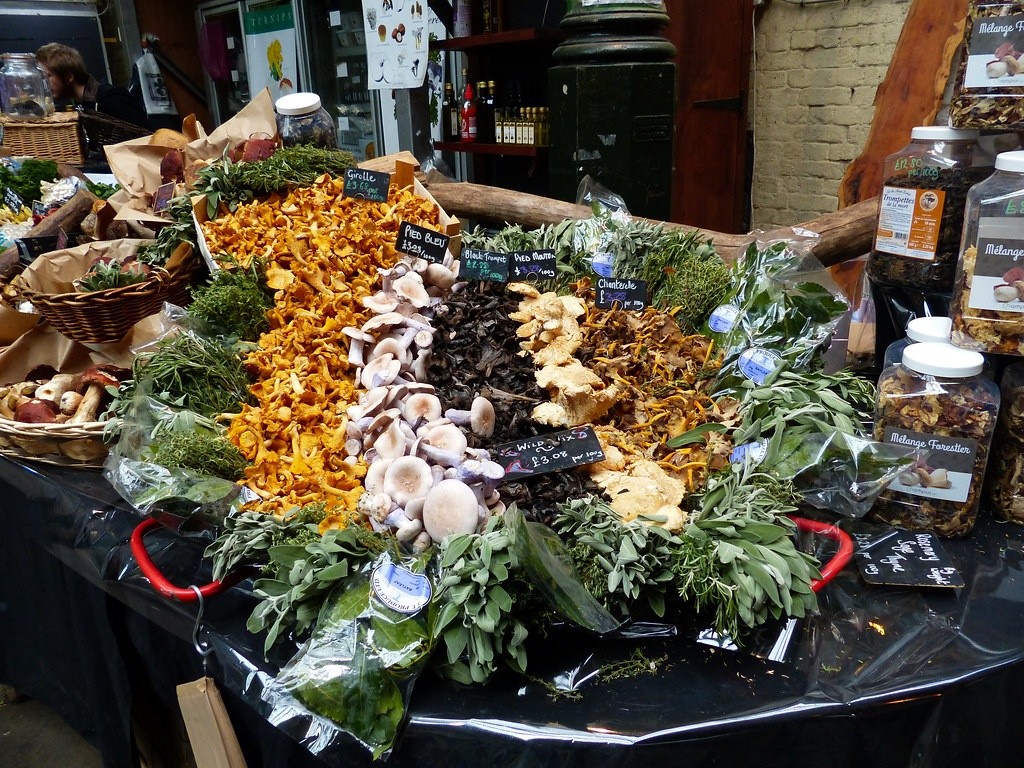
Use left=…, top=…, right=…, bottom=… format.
left=438, top=199, right=882, bottom=691
left=69, top=194, right=438, bottom=756
left=0, top=142, right=360, bottom=213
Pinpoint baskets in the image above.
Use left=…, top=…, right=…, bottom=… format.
left=1, top=380, right=124, bottom=468
left=18, top=239, right=209, bottom=346
left=0, top=109, right=89, bottom=165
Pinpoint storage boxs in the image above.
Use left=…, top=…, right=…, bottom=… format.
left=347, top=12, right=363, bottom=26
left=352, top=29, right=364, bottom=45
left=336, top=30, right=353, bottom=46
left=190, top=160, right=458, bottom=285
left=341, top=13, right=350, bottom=30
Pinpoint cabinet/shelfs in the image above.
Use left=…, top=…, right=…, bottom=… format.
left=426, top=21, right=555, bottom=159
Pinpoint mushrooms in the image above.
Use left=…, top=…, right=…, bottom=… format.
left=0, top=127, right=287, bottom=427
left=986, top=42, right=1024, bottom=78
left=897, top=458, right=951, bottom=489
left=206, top=176, right=735, bottom=553
left=993, top=266, right=1024, bottom=302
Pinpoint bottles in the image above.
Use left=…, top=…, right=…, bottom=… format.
left=946, top=0, right=1024, bottom=132
left=866, top=125, right=995, bottom=290
left=948, top=151, right=1024, bottom=359
left=457, top=69, right=546, bottom=145
left=275, top=92, right=339, bottom=152
left=872, top=317, right=1024, bottom=540
left=442, top=82, right=459, bottom=141
left=0, top=51, right=54, bottom=120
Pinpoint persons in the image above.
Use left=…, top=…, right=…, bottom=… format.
left=34, top=42, right=153, bottom=133
